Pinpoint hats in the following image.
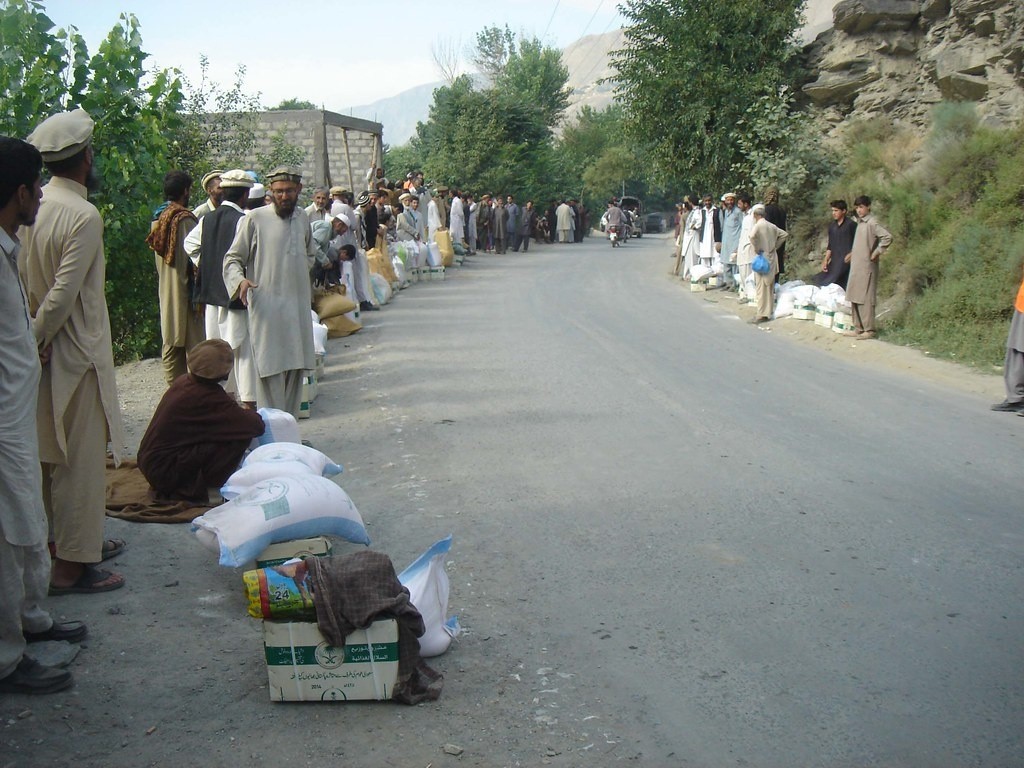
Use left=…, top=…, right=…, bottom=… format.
left=329, top=186, right=347, bottom=194
left=247, top=182, right=265, bottom=198
left=336, top=214, right=351, bottom=227
left=356, top=190, right=368, bottom=201
left=358, top=196, right=370, bottom=206
left=201, top=170, right=225, bottom=193
left=429, top=185, right=490, bottom=201
left=674, top=185, right=779, bottom=214
left=264, top=164, right=303, bottom=184
left=399, top=192, right=410, bottom=200
left=394, top=179, right=402, bottom=187
left=187, top=338, right=235, bottom=379
left=27, top=107, right=92, bottom=161
left=219, top=169, right=257, bottom=188
left=368, top=190, right=378, bottom=196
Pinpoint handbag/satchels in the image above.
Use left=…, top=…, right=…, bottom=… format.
left=750, top=249, right=770, bottom=274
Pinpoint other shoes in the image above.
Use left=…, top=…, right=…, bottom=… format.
left=0, top=654, right=72, bottom=694
left=990, top=398, right=1024, bottom=416
left=857, top=330, right=878, bottom=340
left=23, top=619, right=87, bottom=643
left=843, top=331, right=859, bottom=337
left=716, top=281, right=748, bottom=304
left=478, top=244, right=526, bottom=254
left=747, top=316, right=768, bottom=324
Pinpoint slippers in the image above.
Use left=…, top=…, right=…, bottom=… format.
left=49, top=566, right=126, bottom=594
left=87, top=538, right=126, bottom=567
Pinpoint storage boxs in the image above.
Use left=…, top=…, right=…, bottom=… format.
left=746, top=292, right=778, bottom=305
left=402, top=264, right=446, bottom=288
left=691, top=276, right=723, bottom=292
left=299, top=354, right=326, bottom=420
left=451, top=253, right=466, bottom=266
left=256, top=537, right=333, bottom=569
left=793, top=299, right=856, bottom=334
left=263, top=613, right=401, bottom=702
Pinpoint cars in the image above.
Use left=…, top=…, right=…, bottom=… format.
left=600, top=196, right=667, bottom=237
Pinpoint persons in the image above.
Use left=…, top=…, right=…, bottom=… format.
left=749, top=208, right=789, bottom=324
left=543, top=198, right=590, bottom=243
left=144, top=165, right=535, bottom=420
left=0, top=136, right=89, bottom=694
left=15, top=110, right=127, bottom=596
left=843, top=196, right=893, bottom=340
left=605, top=201, right=638, bottom=233
left=991, top=281, right=1024, bottom=416
left=137, top=338, right=266, bottom=504
left=671, top=186, right=786, bottom=304
left=812, top=200, right=858, bottom=291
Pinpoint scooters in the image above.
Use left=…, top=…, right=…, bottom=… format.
left=608, top=220, right=637, bottom=248
